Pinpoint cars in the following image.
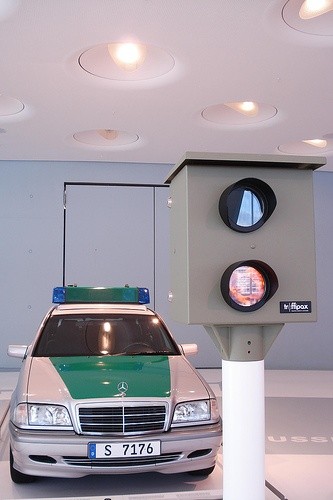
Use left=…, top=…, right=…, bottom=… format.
left=10, top=286, right=223, bottom=483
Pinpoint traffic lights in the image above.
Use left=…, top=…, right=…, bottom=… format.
left=168, top=151, right=326, bottom=323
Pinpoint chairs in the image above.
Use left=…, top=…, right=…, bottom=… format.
left=47, top=320, right=143, bottom=353
